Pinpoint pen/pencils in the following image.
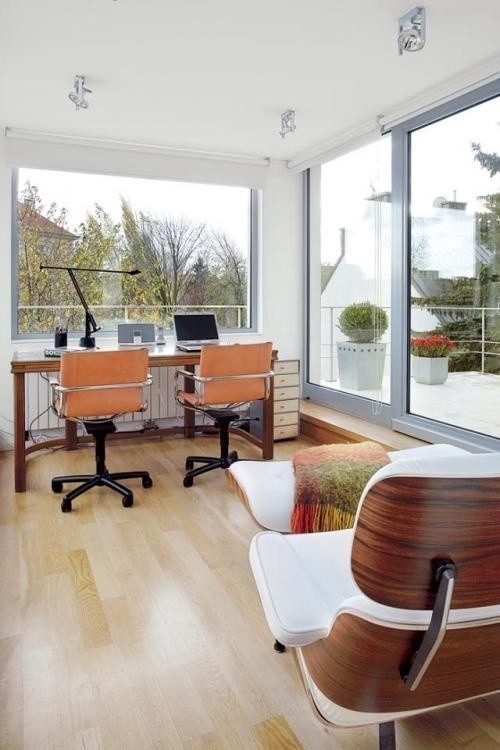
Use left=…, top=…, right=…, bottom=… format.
left=55, top=324, right=68, bottom=334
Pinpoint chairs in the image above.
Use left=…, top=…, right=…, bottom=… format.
left=248, top=453, right=500, bottom=750
left=48, top=347, right=152, bottom=513
left=175, top=341, right=273, bottom=487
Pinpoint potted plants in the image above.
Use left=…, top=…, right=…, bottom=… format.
left=337, top=300, right=389, bottom=390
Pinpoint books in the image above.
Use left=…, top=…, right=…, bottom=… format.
left=44, top=346, right=96, bottom=357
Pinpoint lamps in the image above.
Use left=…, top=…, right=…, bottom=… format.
left=39, top=263, right=142, bottom=347
left=278, top=109, right=297, bottom=137
left=397, top=6, right=426, bottom=55
left=68, top=75, right=93, bottom=110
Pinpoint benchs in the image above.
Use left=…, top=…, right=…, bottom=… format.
left=225, top=444, right=472, bottom=535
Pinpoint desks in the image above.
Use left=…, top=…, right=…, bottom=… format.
left=10, top=349, right=279, bottom=493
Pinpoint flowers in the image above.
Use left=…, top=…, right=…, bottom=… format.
left=409, top=334, right=453, bottom=357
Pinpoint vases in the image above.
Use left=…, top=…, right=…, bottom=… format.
left=412, top=356, right=448, bottom=384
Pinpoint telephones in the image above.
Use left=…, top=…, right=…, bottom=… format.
left=157, top=323, right=166, bottom=344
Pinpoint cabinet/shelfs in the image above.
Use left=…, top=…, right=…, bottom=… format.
left=249, top=359, right=301, bottom=441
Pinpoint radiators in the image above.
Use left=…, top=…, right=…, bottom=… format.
left=25, top=364, right=250, bottom=431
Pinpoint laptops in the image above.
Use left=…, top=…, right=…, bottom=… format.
left=171, top=312, right=220, bottom=351
